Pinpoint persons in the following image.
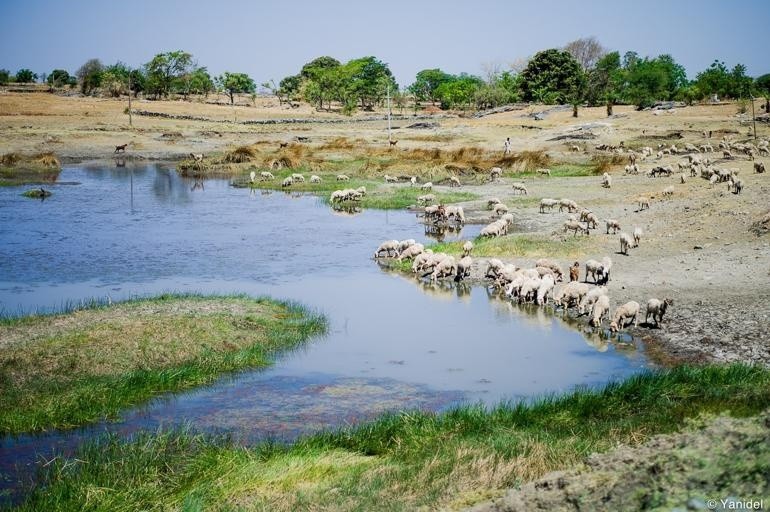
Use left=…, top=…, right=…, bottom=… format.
left=503, top=137, right=511, bottom=154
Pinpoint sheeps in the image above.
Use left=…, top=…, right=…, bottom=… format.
left=537, top=169, right=550, bottom=176
left=422, top=182, right=433, bottom=192
left=633, top=228, right=641, bottom=243
left=330, top=186, right=366, bottom=202
left=600, top=172, right=612, bottom=188
left=113, top=144, right=128, bottom=153
left=298, top=137, right=305, bottom=143
left=620, top=233, right=634, bottom=256
left=646, top=298, right=673, bottom=330
left=479, top=199, right=513, bottom=239
left=487, top=256, right=611, bottom=328
left=638, top=197, right=650, bottom=211
left=425, top=205, right=465, bottom=224
left=375, top=239, right=474, bottom=280
left=384, top=174, right=417, bottom=185
left=337, top=175, right=349, bottom=181
left=450, top=176, right=461, bottom=187
left=190, top=152, right=205, bottom=163
left=625, top=136, right=770, bottom=196
left=596, top=140, right=626, bottom=152
left=417, top=194, right=436, bottom=206
left=609, top=301, right=640, bottom=333
left=389, top=140, right=398, bottom=146
left=539, top=198, right=600, bottom=238
left=512, top=182, right=528, bottom=195
left=607, top=220, right=621, bottom=234
left=280, top=143, right=288, bottom=148
left=489, top=166, right=502, bottom=182
left=572, top=146, right=579, bottom=153
left=249, top=172, right=322, bottom=187
